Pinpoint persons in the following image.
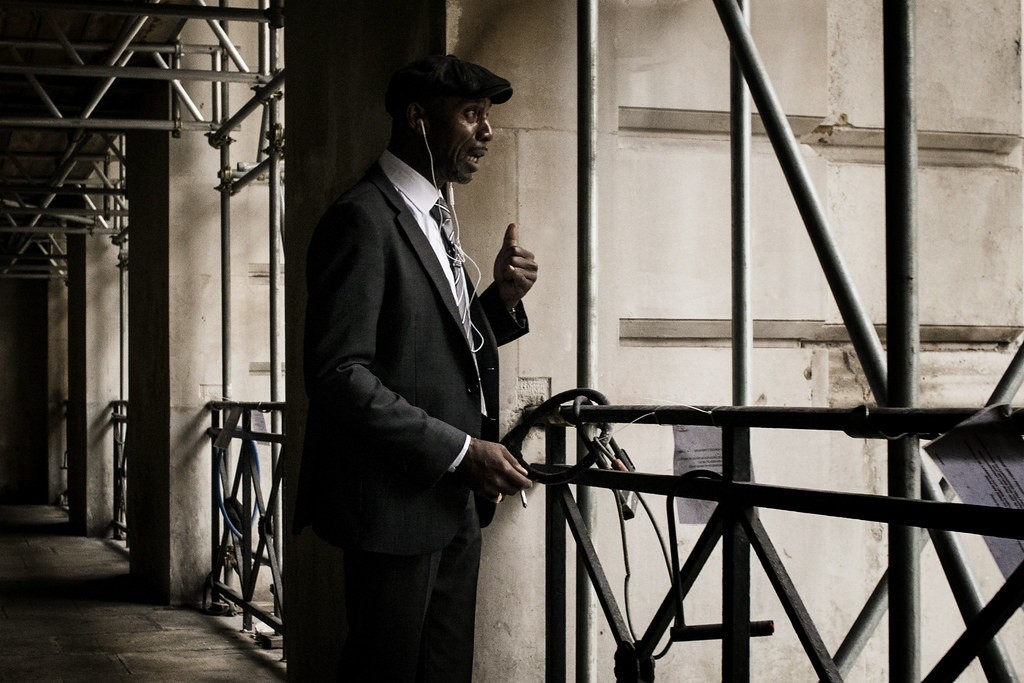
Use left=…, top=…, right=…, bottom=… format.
left=294, top=55, right=539, bottom=682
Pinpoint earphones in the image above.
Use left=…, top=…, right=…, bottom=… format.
left=417, top=119, right=426, bottom=135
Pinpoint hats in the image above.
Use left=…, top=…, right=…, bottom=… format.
left=385, top=55, right=513, bottom=116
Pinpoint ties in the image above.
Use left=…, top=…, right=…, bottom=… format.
left=430, top=199, right=487, bottom=415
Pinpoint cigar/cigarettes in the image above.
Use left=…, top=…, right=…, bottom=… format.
left=517, top=485, right=529, bottom=509
